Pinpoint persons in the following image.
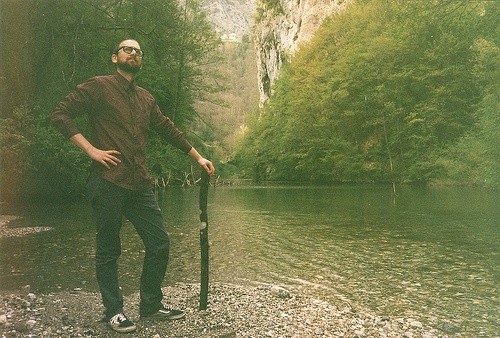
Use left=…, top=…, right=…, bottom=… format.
left=49, top=38, right=216, bottom=332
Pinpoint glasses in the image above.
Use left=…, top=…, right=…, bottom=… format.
left=115, top=46, right=144, bottom=57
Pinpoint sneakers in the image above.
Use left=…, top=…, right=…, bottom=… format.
left=140, top=301, right=186, bottom=321
left=107, top=311, right=138, bottom=334
